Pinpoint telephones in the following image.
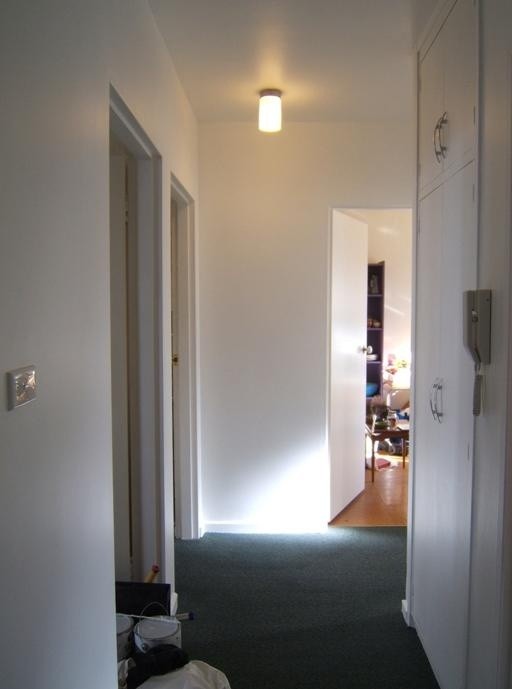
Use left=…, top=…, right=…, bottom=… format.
left=463, top=290, right=493, bottom=364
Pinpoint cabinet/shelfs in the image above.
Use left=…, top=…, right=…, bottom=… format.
left=366, top=260, right=385, bottom=404
left=416, top=0, right=512, bottom=195
left=409, top=157, right=512, bottom=688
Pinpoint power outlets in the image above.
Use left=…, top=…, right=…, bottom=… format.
left=6, top=364, right=39, bottom=411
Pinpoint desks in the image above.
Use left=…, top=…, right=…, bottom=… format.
left=364, top=411, right=409, bottom=484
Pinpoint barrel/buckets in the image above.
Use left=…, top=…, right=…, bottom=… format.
left=116, top=613, right=134, bottom=662
left=132, top=602, right=182, bottom=654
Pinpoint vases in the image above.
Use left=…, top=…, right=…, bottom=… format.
left=370, top=395, right=391, bottom=416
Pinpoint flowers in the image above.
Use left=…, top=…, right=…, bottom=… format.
left=381, top=354, right=408, bottom=396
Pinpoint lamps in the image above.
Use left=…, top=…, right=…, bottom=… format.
left=256, top=88, right=283, bottom=133
